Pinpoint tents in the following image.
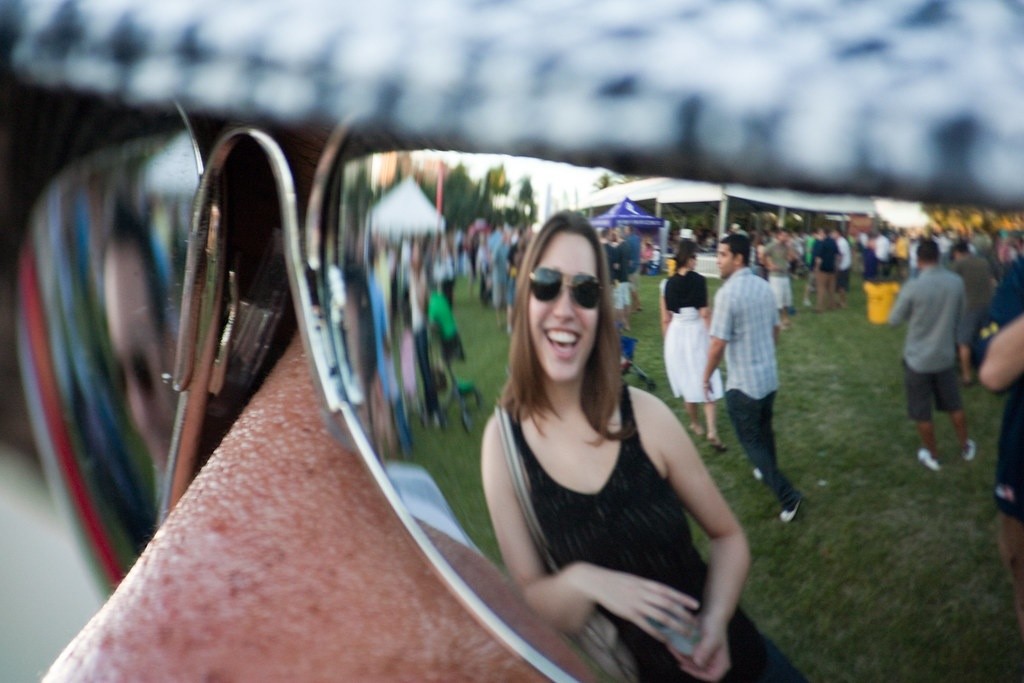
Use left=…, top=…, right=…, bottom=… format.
left=369, top=175, right=446, bottom=240
left=586, top=196, right=666, bottom=243
left=138, top=130, right=198, bottom=196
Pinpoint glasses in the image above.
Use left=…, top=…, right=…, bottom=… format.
left=12, top=114, right=1022, bottom=683
left=528, top=267, right=605, bottom=309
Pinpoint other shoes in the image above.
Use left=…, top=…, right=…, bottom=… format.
left=917, top=448, right=943, bottom=471
left=706, top=433, right=728, bottom=451
left=962, top=439, right=976, bottom=461
left=753, top=468, right=762, bottom=480
left=780, top=497, right=803, bottom=521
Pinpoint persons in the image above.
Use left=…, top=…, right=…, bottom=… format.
left=0, top=0, right=1024, bottom=682
left=17, top=182, right=190, bottom=590
left=329, top=209, right=1023, bottom=683
